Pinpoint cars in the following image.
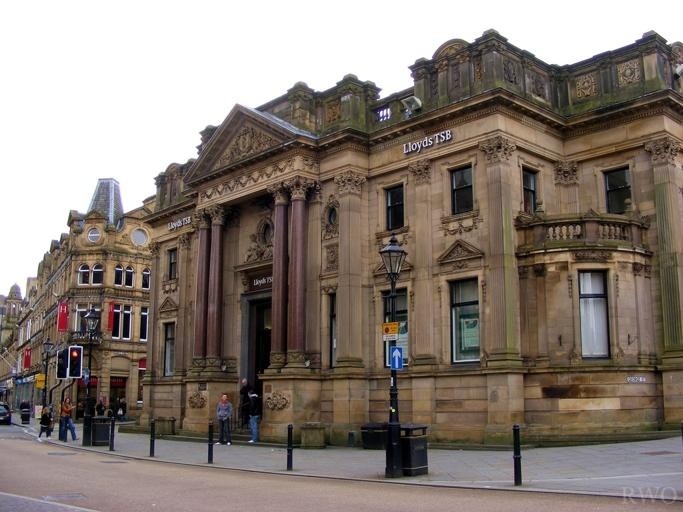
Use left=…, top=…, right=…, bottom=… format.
left=117, top=401, right=143, bottom=421
left=0, top=402, right=11, bottom=425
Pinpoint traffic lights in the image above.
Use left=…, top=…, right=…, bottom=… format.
left=55, top=345, right=83, bottom=379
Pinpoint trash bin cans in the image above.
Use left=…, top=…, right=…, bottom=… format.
left=21, top=409, right=30, bottom=424
left=400, top=425, right=428, bottom=476
left=92, top=416, right=110, bottom=446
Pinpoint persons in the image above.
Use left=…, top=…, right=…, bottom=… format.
left=78, top=397, right=127, bottom=423
left=61, top=397, right=79, bottom=442
left=20, top=400, right=31, bottom=409
left=214, top=393, right=233, bottom=446
left=247, top=389, right=260, bottom=443
left=240, top=378, right=251, bottom=424
left=37, top=407, right=52, bottom=442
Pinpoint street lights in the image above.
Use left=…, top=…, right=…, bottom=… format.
left=82, top=305, right=101, bottom=447
left=378, top=230, right=408, bottom=478
left=42, top=335, right=54, bottom=409
